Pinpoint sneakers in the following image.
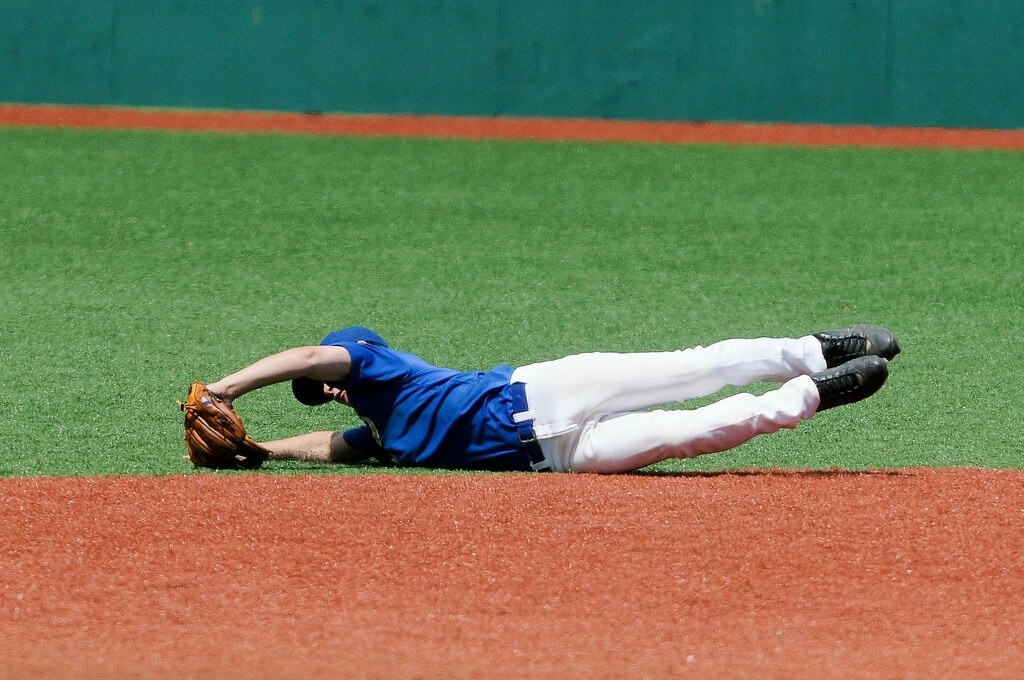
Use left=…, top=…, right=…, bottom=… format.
left=810, top=354, right=889, bottom=412
left=812, top=325, right=901, bottom=368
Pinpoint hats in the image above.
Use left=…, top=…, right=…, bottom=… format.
left=291, top=327, right=389, bottom=405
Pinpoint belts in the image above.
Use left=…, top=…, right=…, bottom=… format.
left=508, top=382, right=553, bottom=473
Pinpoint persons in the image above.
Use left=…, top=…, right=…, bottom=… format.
left=182, top=324, right=902, bottom=474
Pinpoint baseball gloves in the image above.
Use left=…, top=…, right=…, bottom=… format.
left=175, top=381, right=273, bottom=470
